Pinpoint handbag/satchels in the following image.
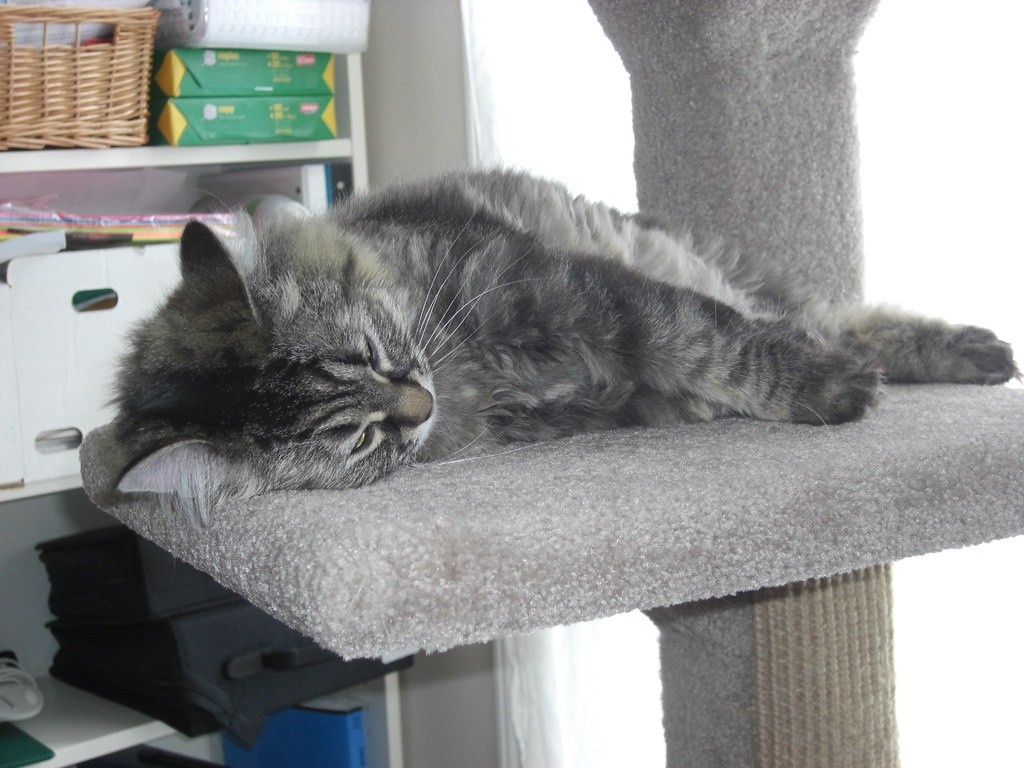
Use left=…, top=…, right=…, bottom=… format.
left=34, top=521, right=413, bottom=750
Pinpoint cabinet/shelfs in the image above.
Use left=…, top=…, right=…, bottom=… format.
left=1, top=1, right=405, bottom=768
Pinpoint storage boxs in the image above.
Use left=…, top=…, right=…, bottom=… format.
left=150, top=48, right=336, bottom=146
left=1, top=241, right=181, bottom=493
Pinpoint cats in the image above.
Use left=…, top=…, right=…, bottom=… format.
left=111, top=160, right=1021, bottom=526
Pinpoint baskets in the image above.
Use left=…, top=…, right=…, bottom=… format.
left=0, top=4, right=160, bottom=149
